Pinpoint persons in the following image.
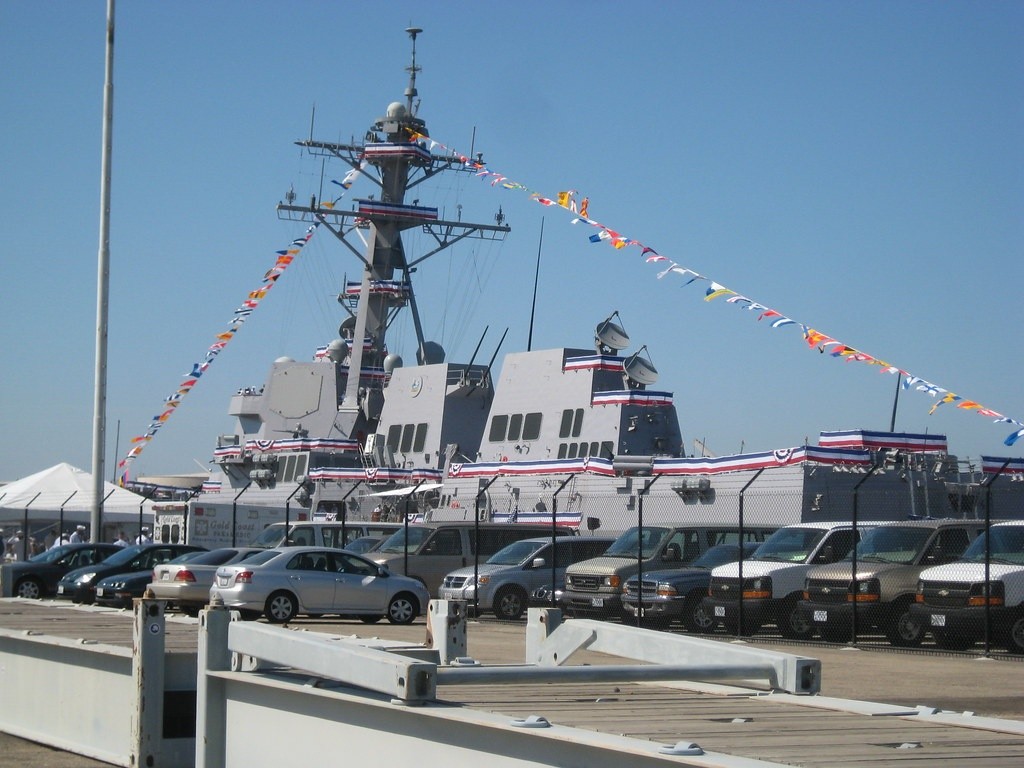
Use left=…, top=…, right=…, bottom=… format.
left=374, top=504, right=383, bottom=514
left=380, top=505, right=390, bottom=522
left=0, top=525, right=152, bottom=563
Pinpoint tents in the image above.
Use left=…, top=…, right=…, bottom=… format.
left=0, top=462, right=158, bottom=523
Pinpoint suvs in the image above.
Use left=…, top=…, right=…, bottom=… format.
left=909, top=517, right=1024, bottom=655
left=704, top=521, right=891, bottom=639
left=796, top=515, right=1012, bottom=648
left=560, top=520, right=786, bottom=647
left=439, top=536, right=622, bottom=621
left=343, top=520, right=577, bottom=604
left=248, top=520, right=406, bottom=561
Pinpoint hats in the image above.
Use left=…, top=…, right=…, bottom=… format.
left=142, top=527, right=149, bottom=531
left=62, top=533, right=69, bottom=537
left=499, top=452, right=501, bottom=454
left=77, top=525, right=86, bottom=530
left=378, top=504, right=381, bottom=506
left=16, top=530, right=23, bottom=533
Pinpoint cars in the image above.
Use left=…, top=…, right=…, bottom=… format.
left=94, top=550, right=210, bottom=610
left=340, top=534, right=392, bottom=559
left=619, top=538, right=763, bottom=637
left=56, top=544, right=211, bottom=608
left=145, top=547, right=269, bottom=618
left=0, top=542, right=134, bottom=600
left=209, top=546, right=431, bottom=625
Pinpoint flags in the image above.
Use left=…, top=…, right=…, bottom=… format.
left=404, top=122, right=1024, bottom=447
left=119, top=156, right=368, bottom=487
